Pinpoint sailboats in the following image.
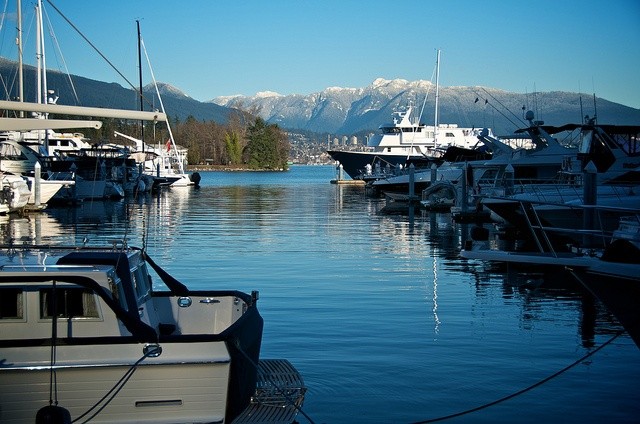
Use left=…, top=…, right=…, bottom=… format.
left=0, top=0, right=202, bottom=217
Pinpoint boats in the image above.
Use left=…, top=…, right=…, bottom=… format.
left=325, top=48, right=640, bottom=353
left=0, top=239, right=306, bottom=424
